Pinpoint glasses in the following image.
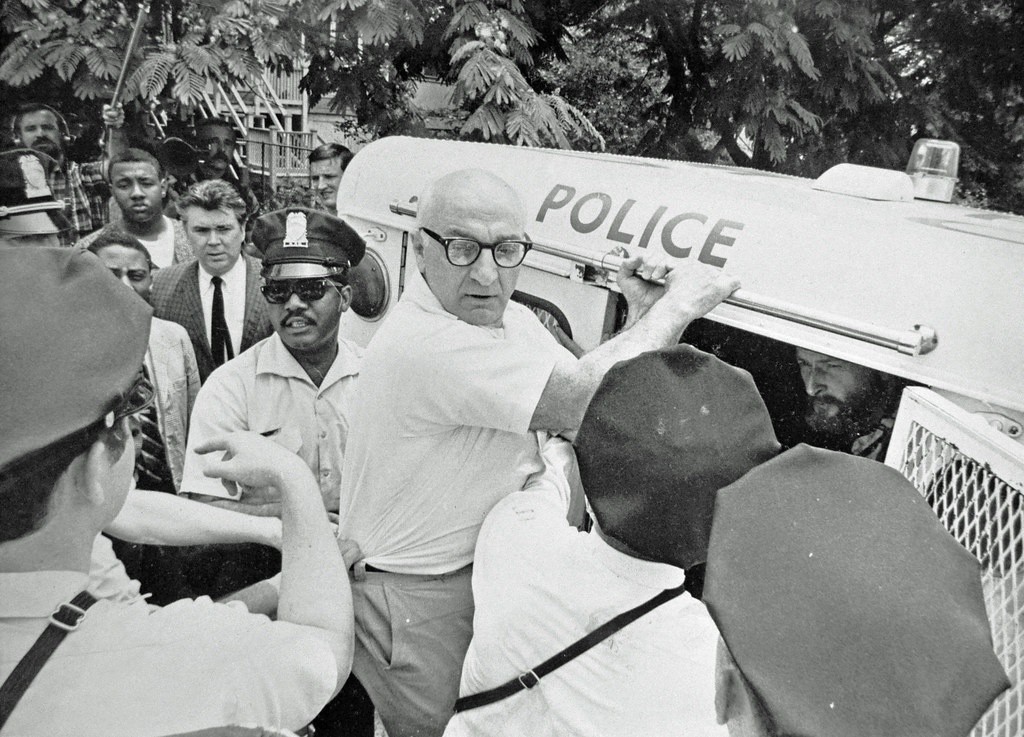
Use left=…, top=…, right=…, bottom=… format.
left=261, top=277, right=334, bottom=305
left=419, top=227, right=532, bottom=268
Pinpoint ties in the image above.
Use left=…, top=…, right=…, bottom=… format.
left=210, top=276, right=234, bottom=368
left=134, top=363, right=176, bottom=485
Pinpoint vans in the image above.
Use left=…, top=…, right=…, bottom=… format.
left=330, top=131, right=1024, bottom=737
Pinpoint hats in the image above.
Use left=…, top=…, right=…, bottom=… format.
left=698, top=440, right=1014, bottom=737
left=0, top=148, right=74, bottom=234
left=572, top=343, right=782, bottom=571
left=0, top=245, right=155, bottom=477
left=251, top=207, right=366, bottom=281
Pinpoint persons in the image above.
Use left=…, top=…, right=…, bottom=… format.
left=795, top=345, right=921, bottom=463
left=0, top=103, right=375, bottom=737
left=438, top=344, right=782, bottom=737
left=338, top=168, right=741, bottom=737
left=703, top=442, right=1012, bottom=737
